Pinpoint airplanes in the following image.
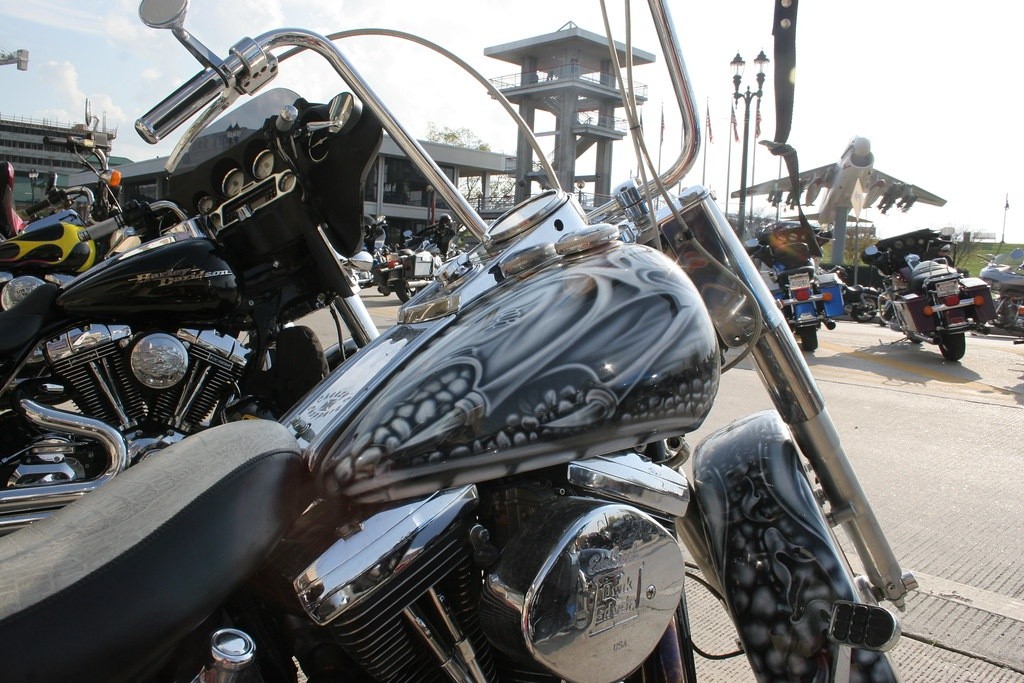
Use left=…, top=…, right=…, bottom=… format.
left=731, top=136, right=948, bottom=230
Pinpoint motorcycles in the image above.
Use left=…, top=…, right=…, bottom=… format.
left=977, top=246, right=1024, bottom=337
left=741, top=215, right=848, bottom=352
left=0, top=1, right=922, bottom=683
left=861, top=226, right=999, bottom=362
